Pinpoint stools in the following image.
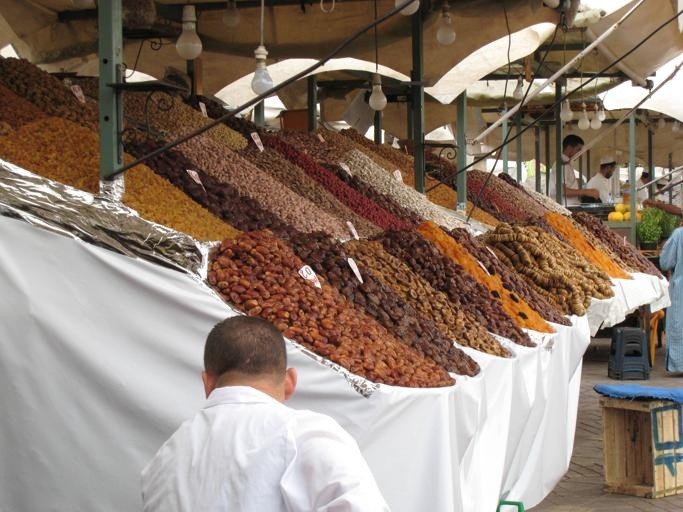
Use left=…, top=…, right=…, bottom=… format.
left=608, top=325, right=651, bottom=380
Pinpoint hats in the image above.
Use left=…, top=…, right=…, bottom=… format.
left=600, top=156, right=614, bottom=165
left=655, top=180, right=669, bottom=185
left=643, top=168, right=649, bottom=173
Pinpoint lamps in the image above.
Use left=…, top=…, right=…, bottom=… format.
left=175, top=2, right=457, bottom=111
left=514, top=27, right=612, bottom=130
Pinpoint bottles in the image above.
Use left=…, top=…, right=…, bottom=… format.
left=623, top=180, right=631, bottom=205
left=607, top=193, right=614, bottom=207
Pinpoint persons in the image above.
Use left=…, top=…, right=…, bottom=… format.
left=584, top=154, right=614, bottom=204
left=635, top=172, right=650, bottom=210
left=653, top=177, right=669, bottom=205
left=548, top=134, right=600, bottom=211
left=137, top=314, right=392, bottom=512
left=658, top=220, right=682, bottom=376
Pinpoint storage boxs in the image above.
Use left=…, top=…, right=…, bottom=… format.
left=598, top=396, right=682, bottom=498
